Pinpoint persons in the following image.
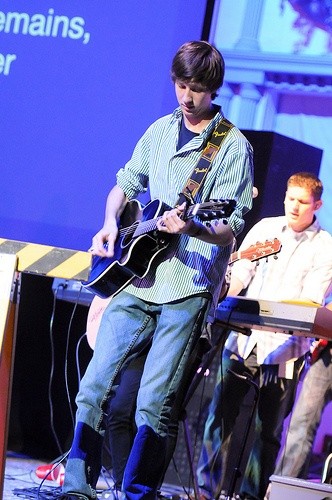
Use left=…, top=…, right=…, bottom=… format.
left=186, top=173, right=332, bottom=500
left=61, top=41, right=254, bottom=500
left=264, top=300, right=332, bottom=500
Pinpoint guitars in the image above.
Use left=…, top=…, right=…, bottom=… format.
left=82, top=199, right=238, bottom=299
left=224, top=240, right=284, bottom=270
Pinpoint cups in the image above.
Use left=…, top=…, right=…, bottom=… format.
left=59, top=473, right=69, bottom=487
left=36, top=463, right=64, bottom=481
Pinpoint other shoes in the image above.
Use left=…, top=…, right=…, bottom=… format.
left=170, top=492, right=207, bottom=500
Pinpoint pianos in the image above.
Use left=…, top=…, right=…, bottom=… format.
left=51, top=278, right=332, bottom=341
